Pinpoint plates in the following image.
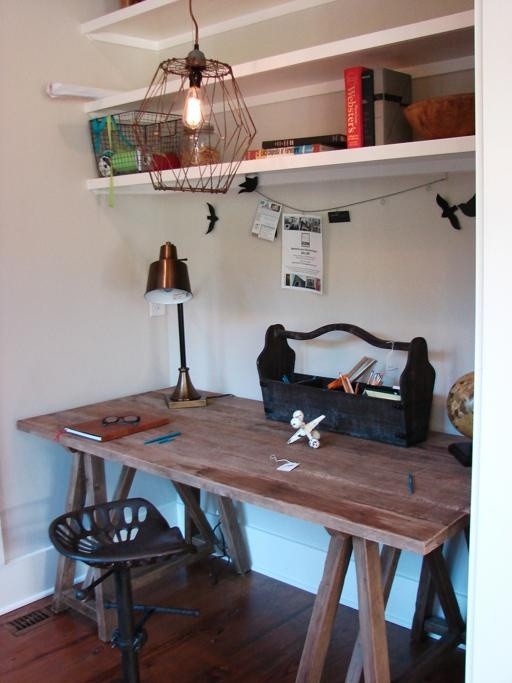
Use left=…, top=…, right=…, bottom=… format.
left=445, top=372, right=473, bottom=440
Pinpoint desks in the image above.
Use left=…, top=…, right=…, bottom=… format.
left=14, top=383, right=470, bottom=682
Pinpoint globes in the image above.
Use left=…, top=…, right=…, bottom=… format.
left=447, top=370, right=474, bottom=467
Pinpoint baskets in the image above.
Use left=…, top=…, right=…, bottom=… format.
left=91, top=111, right=182, bottom=176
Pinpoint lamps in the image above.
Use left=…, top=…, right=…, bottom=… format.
left=143, top=240, right=207, bottom=409
left=133, top=0, right=256, bottom=193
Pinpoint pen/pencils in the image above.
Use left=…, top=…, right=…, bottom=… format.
left=339, top=370, right=360, bottom=395
left=281, top=374, right=290, bottom=384
left=144, top=431, right=181, bottom=445
left=367, top=370, right=385, bottom=386
left=408, top=473, right=413, bottom=497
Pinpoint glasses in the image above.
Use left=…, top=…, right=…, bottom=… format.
left=102, top=415, right=141, bottom=426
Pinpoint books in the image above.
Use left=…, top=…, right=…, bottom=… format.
left=359, top=68, right=375, bottom=147
left=374, top=66, right=413, bottom=146
left=245, top=144, right=335, bottom=162
left=342, top=64, right=362, bottom=148
left=61, top=410, right=171, bottom=443
left=260, top=132, right=346, bottom=148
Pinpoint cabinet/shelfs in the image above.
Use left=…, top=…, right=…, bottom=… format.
left=76, top=1, right=475, bottom=194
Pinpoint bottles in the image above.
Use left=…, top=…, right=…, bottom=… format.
left=178, top=123, right=222, bottom=167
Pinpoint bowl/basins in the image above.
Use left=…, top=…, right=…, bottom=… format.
left=109, top=149, right=178, bottom=174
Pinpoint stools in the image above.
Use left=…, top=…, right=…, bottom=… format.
left=50, top=498, right=200, bottom=681
